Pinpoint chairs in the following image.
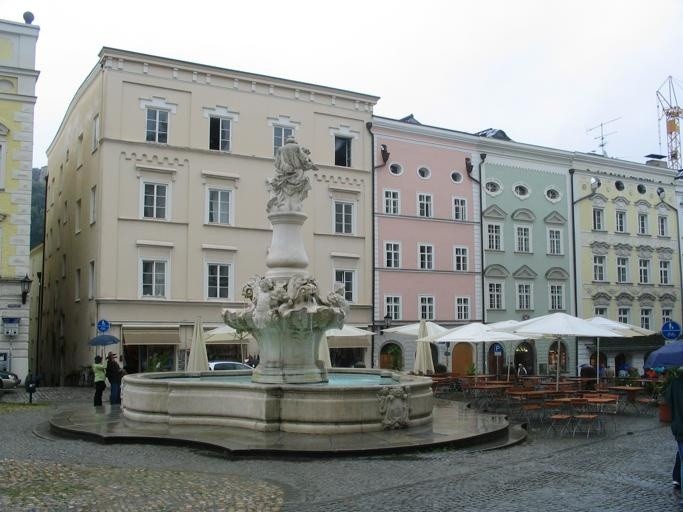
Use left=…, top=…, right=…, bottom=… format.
left=430, top=372, right=663, bottom=440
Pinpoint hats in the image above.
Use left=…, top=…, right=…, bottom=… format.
left=94, top=356, right=102, bottom=363
left=106, top=351, right=116, bottom=359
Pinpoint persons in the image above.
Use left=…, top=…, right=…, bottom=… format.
left=265, top=136, right=319, bottom=211
left=334, top=283, right=346, bottom=296
left=665, top=372, right=682, bottom=496
left=93, top=355, right=106, bottom=408
left=258, top=277, right=274, bottom=293
left=105, top=351, right=123, bottom=405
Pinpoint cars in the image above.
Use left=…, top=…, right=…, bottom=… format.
left=0, top=371, right=19, bottom=389
left=208, top=361, right=254, bottom=370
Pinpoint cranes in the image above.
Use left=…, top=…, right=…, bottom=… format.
left=656, top=76, right=683, bottom=169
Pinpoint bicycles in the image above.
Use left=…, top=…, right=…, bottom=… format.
left=78, top=365, right=94, bottom=387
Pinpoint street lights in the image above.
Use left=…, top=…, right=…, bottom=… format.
left=569, top=169, right=598, bottom=377
left=465, top=153, right=486, bottom=374
left=366, top=122, right=389, bottom=368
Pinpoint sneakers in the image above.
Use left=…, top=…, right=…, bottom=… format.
left=672, top=480, right=680, bottom=485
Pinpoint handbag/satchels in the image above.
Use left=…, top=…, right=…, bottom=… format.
left=660, top=401, right=673, bottom=422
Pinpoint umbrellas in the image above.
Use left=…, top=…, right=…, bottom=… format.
left=186, top=315, right=210, bottom=372
left=643, top=340, right=683, bottom=369
left=325, top=325, right=377, bottom=348
left=87, top=335, right=121, bottom=351
left=203, top=323, right=258, bottom=365
left=380, top=312, right=658, bottom=391
